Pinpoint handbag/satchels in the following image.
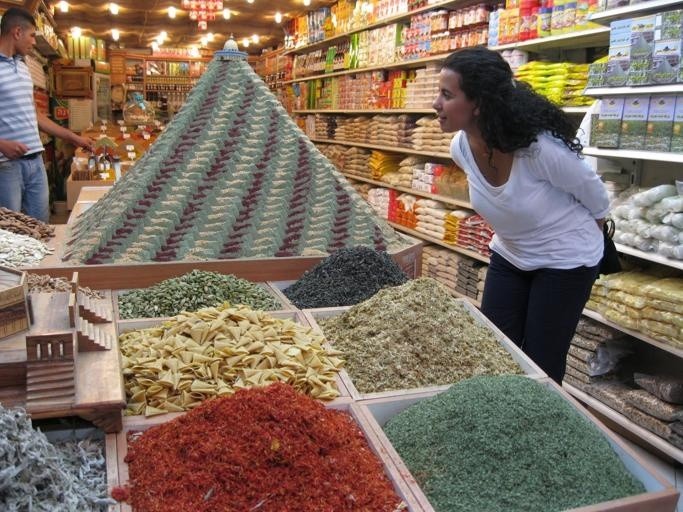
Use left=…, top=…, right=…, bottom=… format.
left=598, top=219, right=622, bottom=275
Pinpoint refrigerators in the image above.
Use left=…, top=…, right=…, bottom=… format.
left=69, top=59, right=111, bottom=130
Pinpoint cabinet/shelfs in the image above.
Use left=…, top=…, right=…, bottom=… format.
left=108, top=0, right=683, bottom=464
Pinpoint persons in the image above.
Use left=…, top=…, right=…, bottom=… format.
left=0, top=8, right=95, bottom=227
left=433, top=47, right=612, bottom=388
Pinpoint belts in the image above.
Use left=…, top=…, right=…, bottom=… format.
left=20, top=151, right=42, bottom=160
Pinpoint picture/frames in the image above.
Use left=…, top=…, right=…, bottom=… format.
left=52, top=66, right=93, bottom=98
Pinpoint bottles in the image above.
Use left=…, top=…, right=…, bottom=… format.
left=295, top=40, right=350, bottom=79
left=72, top=149, right=112, bottom=181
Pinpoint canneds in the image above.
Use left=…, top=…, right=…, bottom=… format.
left=428, top=2, right=504, bottom=57
